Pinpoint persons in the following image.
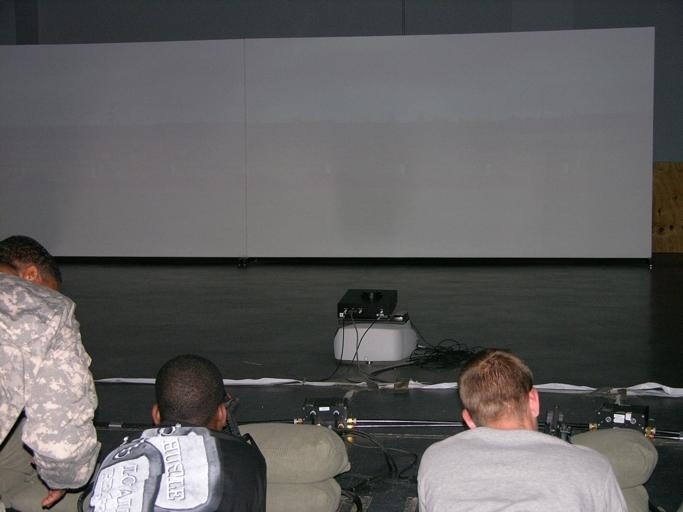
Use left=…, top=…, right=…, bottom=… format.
left=79, top=353, right=267, bottom=512
left=415, top=347, right=629, bottom=512
left=0, top=234, right=101, bottom=512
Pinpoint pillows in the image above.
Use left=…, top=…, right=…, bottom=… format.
left=238, top=423, right=351, bottom=481
left=563, top=429, right=658, bottom=486
left=621, top=485, right=648, bottom=510
left=265, top=479, right=342, bottom=511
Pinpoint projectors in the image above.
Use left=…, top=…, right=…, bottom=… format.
left=336, top=289, right=398, bottom=320
